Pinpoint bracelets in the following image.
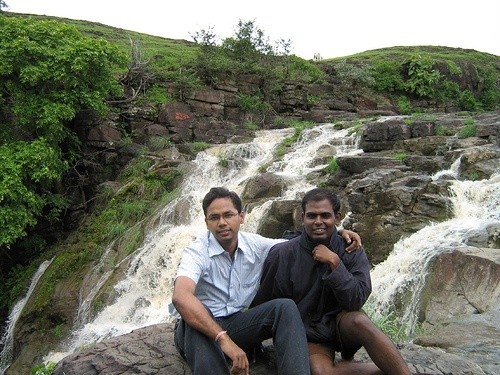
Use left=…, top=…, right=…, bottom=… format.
left=215, top=331, right=227, bottom=342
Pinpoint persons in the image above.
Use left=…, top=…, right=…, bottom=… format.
left=249, top=189, right=412, bottom=375
left=171, top=187, right=363, bottom=375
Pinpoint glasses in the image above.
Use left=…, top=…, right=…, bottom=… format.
left=206, top=211, right=240, bottom=223
